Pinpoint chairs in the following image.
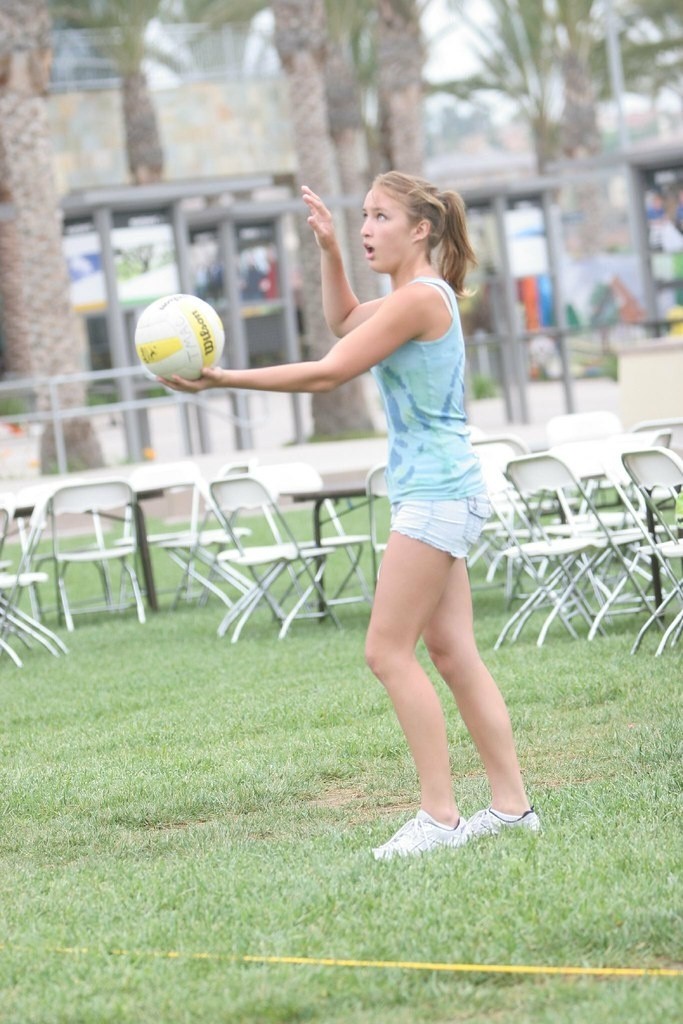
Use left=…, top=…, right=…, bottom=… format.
left=0, top=407, right=683, bottom=668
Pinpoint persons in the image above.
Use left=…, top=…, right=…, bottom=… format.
left=157, top=171, right=541, bottom=859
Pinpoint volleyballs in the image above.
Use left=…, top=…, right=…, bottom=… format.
left=134, top=293, right=227, bottom=384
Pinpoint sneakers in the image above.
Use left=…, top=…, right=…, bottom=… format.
left=464, top=805, right=541, bottom=835
left=370, top=808, right=468, bottom=859
